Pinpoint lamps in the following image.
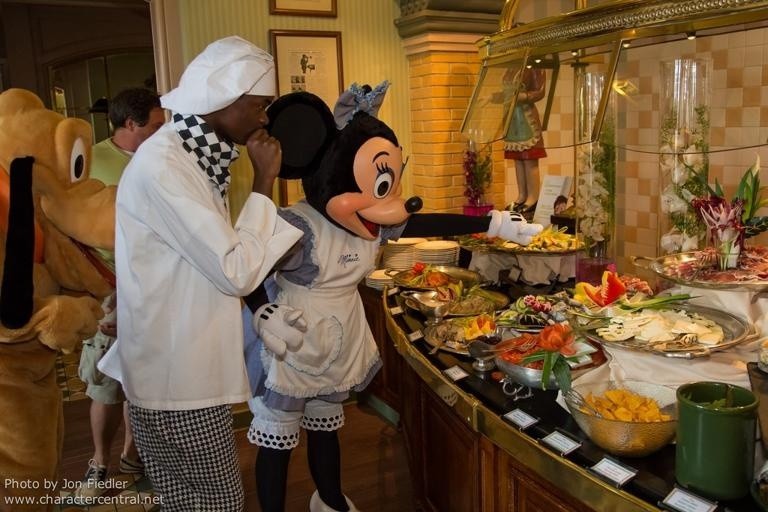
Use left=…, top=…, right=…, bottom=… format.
left=87, top=97, right=111, bottom=114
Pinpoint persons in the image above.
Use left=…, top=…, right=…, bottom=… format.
left=99, top=36, right=306, bottom=512
left=83, top=86, right=166, bottom=503
left=478, top=20, right=546, bottom=222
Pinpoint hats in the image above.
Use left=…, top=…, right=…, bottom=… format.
left=160, top=35, right=277, bottom=115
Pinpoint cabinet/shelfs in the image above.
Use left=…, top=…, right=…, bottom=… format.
left=356, top=279, right=406, bottom=423
left=395, top=372, right=597, bottom=512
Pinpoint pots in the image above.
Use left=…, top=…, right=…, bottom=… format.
left=493, top=338, right=599, bottom=391
left=383, top=262, right=510, bottom=320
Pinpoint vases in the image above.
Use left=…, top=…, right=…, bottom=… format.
left=462, top=204, right=493, bottom=218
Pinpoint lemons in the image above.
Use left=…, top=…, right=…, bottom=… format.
left=574, top=282, right=595, bottom=306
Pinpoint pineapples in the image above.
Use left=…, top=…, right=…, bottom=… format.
left=579, top=389, right=673, bottom=456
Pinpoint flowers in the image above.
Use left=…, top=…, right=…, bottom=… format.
left=455, top=140, right=497, bottom=203
left=578, top=103, right=618, bottom=259
left=659, top=98, right=709, bottom=254
left=689, top=160, right=768, bottom=262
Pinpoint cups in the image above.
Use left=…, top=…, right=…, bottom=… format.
left=674, top=379, right=757, bottom=498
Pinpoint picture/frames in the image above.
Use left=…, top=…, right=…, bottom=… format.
left=266, top=0, right=342, bottom=19
left=280, top=172, right=306, bottom=209
left=268, top=28, right=344, bottom=112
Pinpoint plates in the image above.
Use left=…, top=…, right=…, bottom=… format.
left=366, top=236, right=456, bottom=291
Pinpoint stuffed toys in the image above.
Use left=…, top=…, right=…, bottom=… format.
left=240, top=78, right=544, bottom=511
left=0, top=86, right=117, bottom=512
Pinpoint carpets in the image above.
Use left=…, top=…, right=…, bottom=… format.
left=55, top=351, right=98, bottom=403
left=49, top=469, right=163, bottom=512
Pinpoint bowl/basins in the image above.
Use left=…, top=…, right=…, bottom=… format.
left=559, top=379, right=678, bottom=453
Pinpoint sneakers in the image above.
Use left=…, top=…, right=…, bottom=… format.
left=74, top=459, right=108, bottom=505
left=119, top=454, right=144, bottom=474
